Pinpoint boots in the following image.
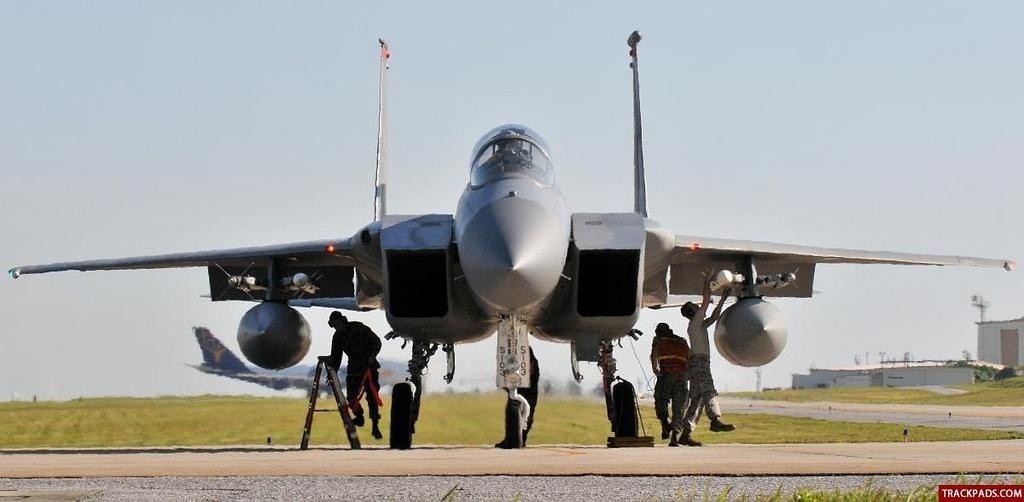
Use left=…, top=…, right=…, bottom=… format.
left=661, top=417, right=679, bottom=447
left=353, top=413, right=383, bottom=438
left=678, top=428, right=701, bottom=446
left=709, top=417, right=735, bottom=432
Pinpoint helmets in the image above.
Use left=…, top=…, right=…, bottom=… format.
left=656, top=323, right=673, bottom=334
left=328, top=311, right=347, bottom=327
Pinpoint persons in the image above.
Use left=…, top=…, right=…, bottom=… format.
left=650, top=324, right=691, bottom=446
left=679, top=273, right=736, bottom=446
left=517, top=347, right=539, bottom=447
left=325, top=311, right=383, bottom=438
